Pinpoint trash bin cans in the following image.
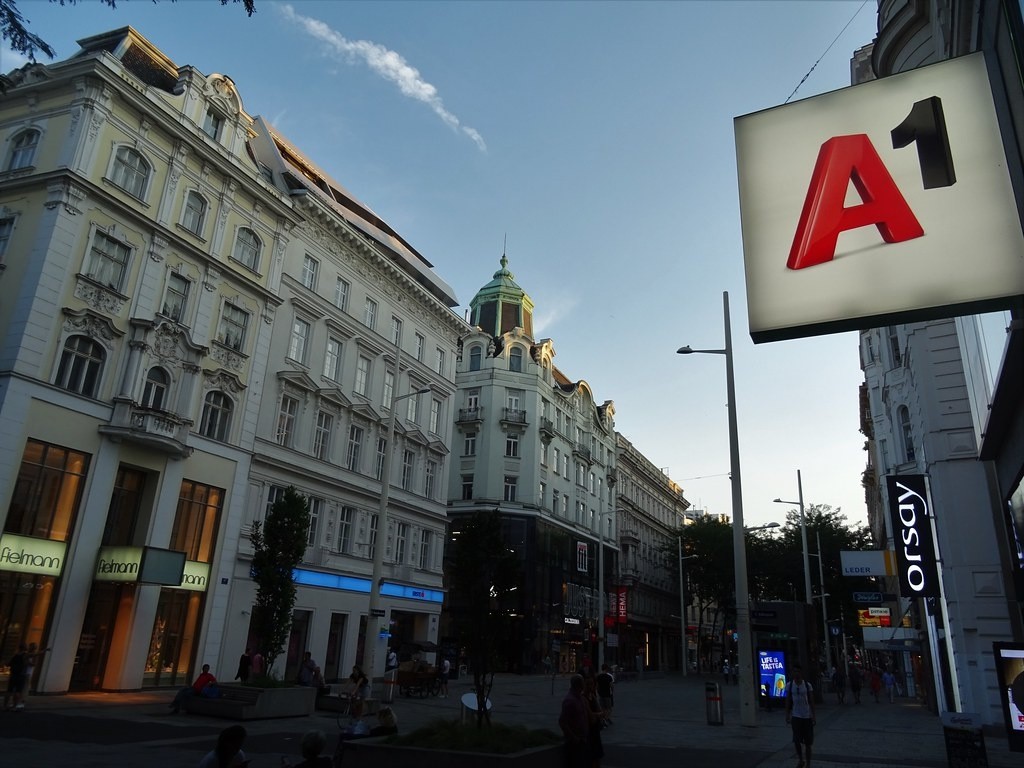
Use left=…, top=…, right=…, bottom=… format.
left=461, top=692, right=493, bottom=725
left=706, top=681, right=723, bottom=726
left=382, top=668, right=399, bottom=704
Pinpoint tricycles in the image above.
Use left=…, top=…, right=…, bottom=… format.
left=400, top=668, right=441, bottom=699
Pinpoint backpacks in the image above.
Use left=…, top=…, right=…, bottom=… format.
left=362, top=670, right=373, bottom=682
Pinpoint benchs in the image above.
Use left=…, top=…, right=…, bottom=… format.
left=318, top=693, right=378, bottom=716
left=185, top=683, right=265, bottom=720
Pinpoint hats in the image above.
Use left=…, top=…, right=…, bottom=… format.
left=258, top=649, right=264, bottom=656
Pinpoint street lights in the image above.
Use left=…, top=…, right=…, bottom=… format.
left=802, top=529, right=831, bottom=681
left=598, top=491, right=626, bottom=671
left=773, top=470, right=816, bottom=707
left=679, top=536, right=699, bottom=679
left=362, top=350, right=431, bottom=703
left=677, top=345, right=750, bottom=727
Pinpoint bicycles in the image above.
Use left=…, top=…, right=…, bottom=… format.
left=336, top=691, right=368, bottom=731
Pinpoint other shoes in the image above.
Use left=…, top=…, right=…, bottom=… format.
left=871, top=691, right=877, bottom=696
left=3, top=705, right=9, bottom=711
left=899, top=693, right=904, bottom=696
left=839, top=699, right=845, bottom=703
left=796, top=760, right=810, bottom=768
left=167, top=703, right=181, bottom=715
left=855, top=699, right=861, bottom=704
left=9, top=703, right=14, bottom=708
left=606, top=717, right=612, bottom=724
left=15, top=704, right=25, bottom=711
left=600, top=717, right=607, bottom=729
left=439, top=694, right=448, bottom=698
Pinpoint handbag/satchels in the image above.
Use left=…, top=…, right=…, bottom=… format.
left=202, top=681, right=220, bottom=698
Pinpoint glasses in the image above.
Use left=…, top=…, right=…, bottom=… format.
left=797, top=687, right=800, bottom=696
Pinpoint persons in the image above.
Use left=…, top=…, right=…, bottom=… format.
left=6, top=642, right=50, bottom=708
left=200, top=724, right=248, bottom=768
left=438, top=656, right=450, bottom=698
left=785, top=665, right=817, bottom=768
left=835, top=670, right=846, bottom=704
left=850, top=670, right=862, bottom=703
left=868, top=669, right=895, bottom=704
left=542, top=655, right=551, bottom=679
left=597, top=665, right=614, bottom=726
left=235, top=647, right=252, bottom=682
left=280, top=729, right=333, bottom=768
left=561, top=655, right=567, bottom=677
left=344, top=666, right=370, bottom=712
left=174, top=664, right=216, bottom=709
left=254, top=647, right=269, bottom=676
left=723, top=663, right=729, bottom=685
left=301, top=652, right=320, bottom=686
left=731, top=666, right=737, bottom=685
left=558, top=674, right=608, bottom=768
